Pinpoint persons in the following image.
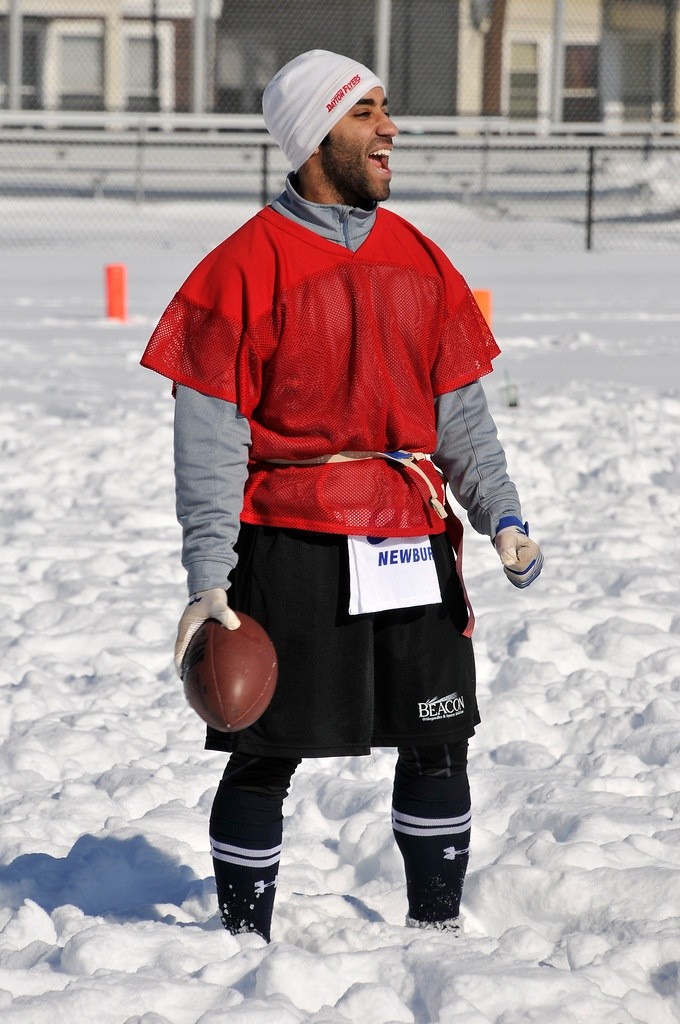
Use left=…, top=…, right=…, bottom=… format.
left=138, top=49, right=543, bottom=942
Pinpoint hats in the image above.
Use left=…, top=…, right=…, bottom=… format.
left=261, top=49, right=386, bottom=175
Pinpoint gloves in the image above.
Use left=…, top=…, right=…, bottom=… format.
left=493, top=525, right=542, bottom=590
left=173, top=588, right=241, bottom=681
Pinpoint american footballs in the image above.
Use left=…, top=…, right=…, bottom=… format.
left=182, top=609, right=279, bottom=733
